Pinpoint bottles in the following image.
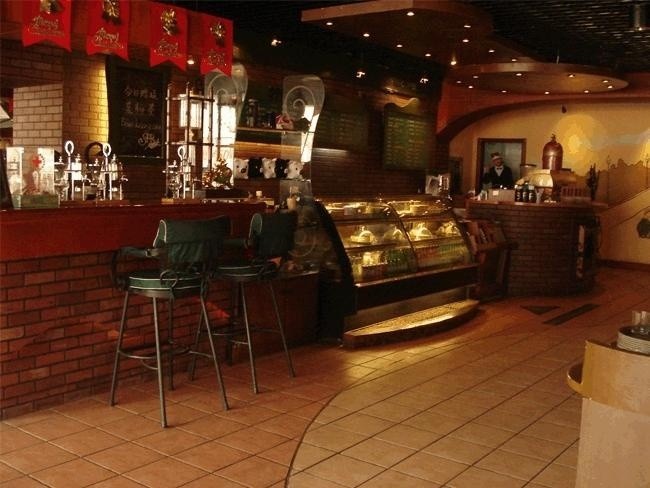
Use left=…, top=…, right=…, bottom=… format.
left=514, top=185, right=539, bottom=204
left=55, top=153, right=124, bottom=203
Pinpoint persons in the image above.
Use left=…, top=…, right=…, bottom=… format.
left=482, top=152, right=514, bottom=189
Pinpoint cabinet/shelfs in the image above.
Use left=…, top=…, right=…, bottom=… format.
left=292, top=196, right=480, bottom=344
left=230, top=127, right=311, bottom=184
left=161, top=82, right=217, bottom=205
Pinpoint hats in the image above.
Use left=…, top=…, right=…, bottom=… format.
left=490, top=152, right=501, bottom=160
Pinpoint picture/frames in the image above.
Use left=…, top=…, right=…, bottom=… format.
left=475, top=138, right=526, bottom=196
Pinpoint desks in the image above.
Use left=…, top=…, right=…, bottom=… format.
left=565, top=338, right=650, bottom=487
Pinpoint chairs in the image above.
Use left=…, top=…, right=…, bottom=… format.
left=108, top=209, right=300, bottom=427
